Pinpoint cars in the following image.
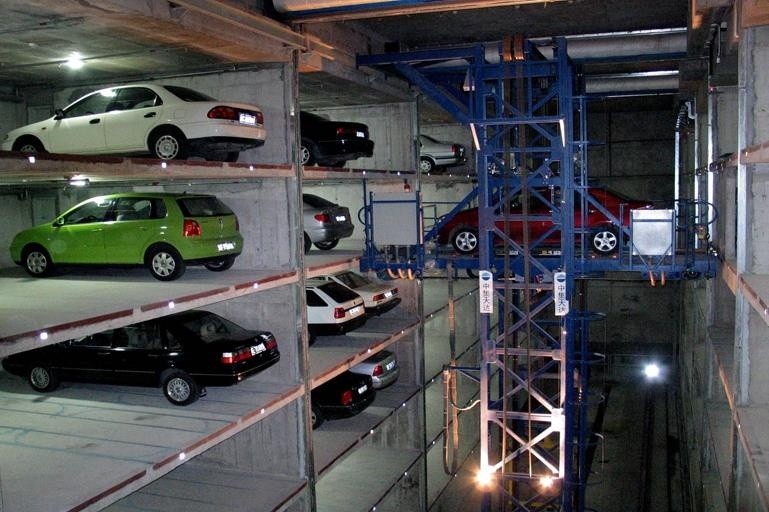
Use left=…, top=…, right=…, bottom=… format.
left=303, top=194, right=353, bottom=256
left=306, top=270, right=400, bottom=347
left=1, top=83, right=267, bottom=161
left=434, top=188, right=655, bottom=255
left=2, top=311, right=279, bottom=405
left=300, top=110, right=373, bottom=168
left=10, top=192, right=243, bottom=281
left=311, top=350, right=400, bottom=431
left=419, top=134, right=464, bottom=175
